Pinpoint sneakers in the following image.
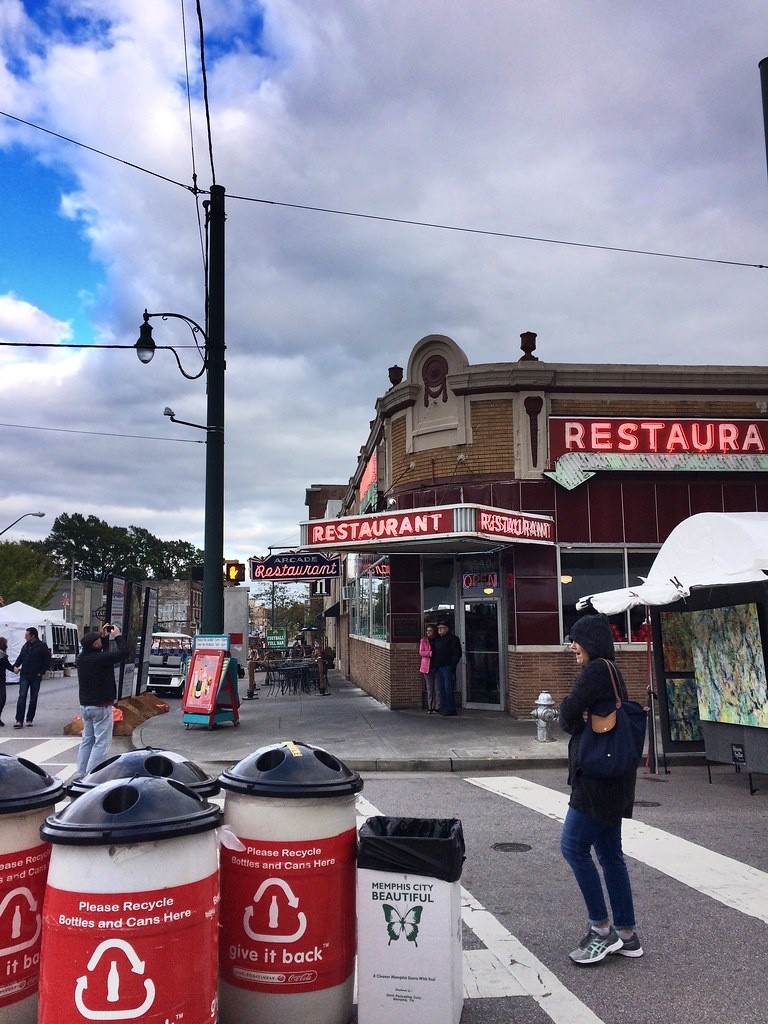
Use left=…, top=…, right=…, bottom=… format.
left=610, top=931, right=644, bottom=957
left=569, top=926, right=624, bottom=965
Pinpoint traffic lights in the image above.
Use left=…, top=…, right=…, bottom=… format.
left=226, top=561, right=245, bottom=584
left=191, top=566, right=203, bottom=583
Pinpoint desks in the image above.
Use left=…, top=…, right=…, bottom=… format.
left=276, top=664, right=309, bottom=694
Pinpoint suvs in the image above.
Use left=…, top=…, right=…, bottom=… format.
left=249, top=635, right=261, bottom=648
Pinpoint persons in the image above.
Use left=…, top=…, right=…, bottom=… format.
left=558, top=612, right=644, bottom=964
left=419, top=622, right=462, bottom=716
left=10, top=627, right=49, bottom=728
left=75, top=623, right=129, bottom=777
left=0, top=637, right=19, bottom=726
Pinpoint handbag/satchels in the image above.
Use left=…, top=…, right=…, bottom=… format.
left=576, top=659, right=647, bottom=773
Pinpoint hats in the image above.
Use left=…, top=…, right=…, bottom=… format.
left=436, top=621, right=450, bottom=629
left=80, top=631, right=102, bottom=646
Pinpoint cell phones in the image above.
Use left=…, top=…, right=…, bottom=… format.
left=106, top=626, right=114, bottom=633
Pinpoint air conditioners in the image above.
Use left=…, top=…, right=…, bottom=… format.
left=342, top=585, right=355, bottom=600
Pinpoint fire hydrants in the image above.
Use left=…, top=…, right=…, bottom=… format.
left=531, top=690, right=560, bottom=742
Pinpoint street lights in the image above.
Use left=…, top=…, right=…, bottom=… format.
left=156, top=581, right=178, bottom=632
left=69, top=539, right=88, bottom=625
left=134, top=311, right=226, bottom=636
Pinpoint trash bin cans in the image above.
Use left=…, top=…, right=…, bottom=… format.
left=37, top=773, right=224, bottom=1024
left=0, top=752, right=66, bottom=1024
left=218, top=741, right=365, bottom=1024
left=356, top=815, right=468, bottom=1024
left=63, top=746, right=222, bottom=806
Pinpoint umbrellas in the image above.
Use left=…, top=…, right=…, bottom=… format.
left=298, top=626, right=326, bottom=645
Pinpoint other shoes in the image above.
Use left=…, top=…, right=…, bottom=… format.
left=12, top=721, right=23, bottom=728
left=0, top=720, right=4, bottom=726
left=427, top=707, right=458, bottom=716
left=25, top=720, right=33, bottom=726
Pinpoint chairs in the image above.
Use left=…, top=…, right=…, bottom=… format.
left=266, top=655, right=331, bottom=695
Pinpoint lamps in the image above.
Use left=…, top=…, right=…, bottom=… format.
left=484, top=588, right=494, bottom=594
left=383, top=485, right=399, bottom=509
left=560, top=575, right=572, bottom=584
